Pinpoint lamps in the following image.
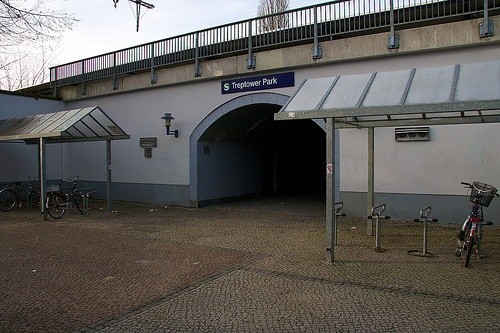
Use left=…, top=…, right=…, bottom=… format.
left=160, top=111, right=179, bottom=138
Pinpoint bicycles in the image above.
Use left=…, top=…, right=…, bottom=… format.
left=456, top=181, right=500, bottom=267
left=45, top=176, right=92, bottom=219
left=0, top=175, right=41, bottom=211
